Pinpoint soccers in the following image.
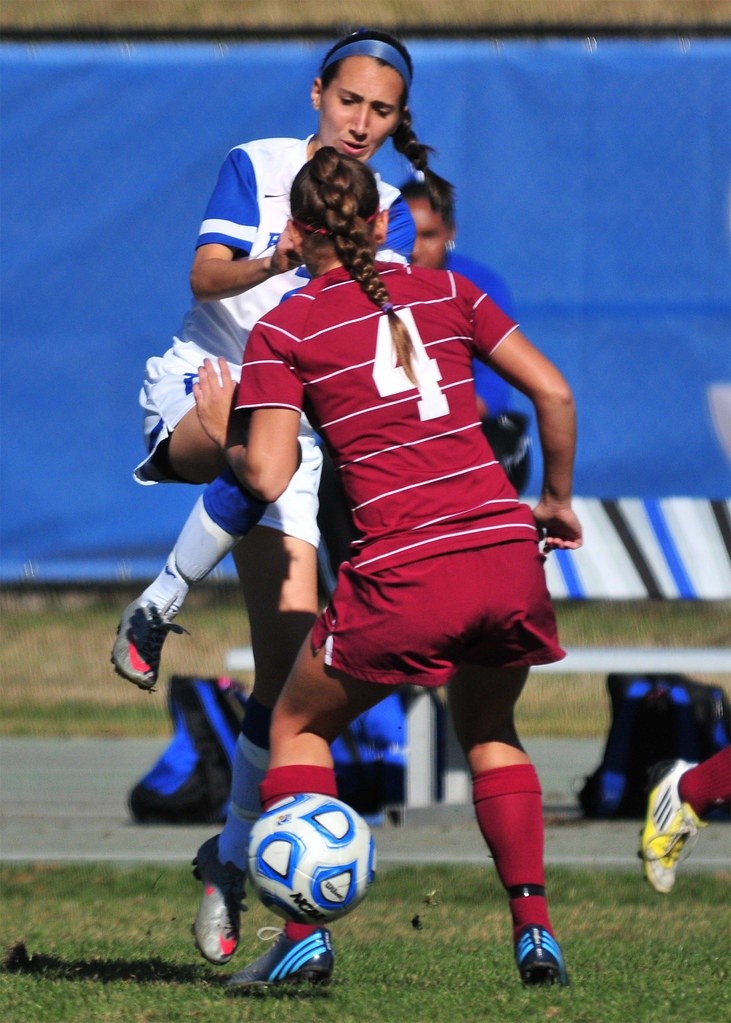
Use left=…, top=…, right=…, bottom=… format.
left=245, top=793, right=376, bottom=923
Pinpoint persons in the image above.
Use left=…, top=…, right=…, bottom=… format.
left=188, top=145, right=587, bottom=1000
left=104, top=27, right=462, bottom=963
left=392, top=170, right=532, bottom=501
left=639, top=742, right=730, bottom=895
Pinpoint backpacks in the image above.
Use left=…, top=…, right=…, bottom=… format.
left=576, top=673, right=731, bottom=822
left=330, top=692, right=405, bottom=823
left=127, top=674, right=246, bottom=825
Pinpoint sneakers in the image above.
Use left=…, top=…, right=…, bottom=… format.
left=514, top=924, right=570, bottom=990
left=639, top=760, right=707, bottom=894
left=224, top=926, right=333, bottom=996
left=111, top=598, right=178, bottom=690
left=192, top=835, right=247, bottom=965
